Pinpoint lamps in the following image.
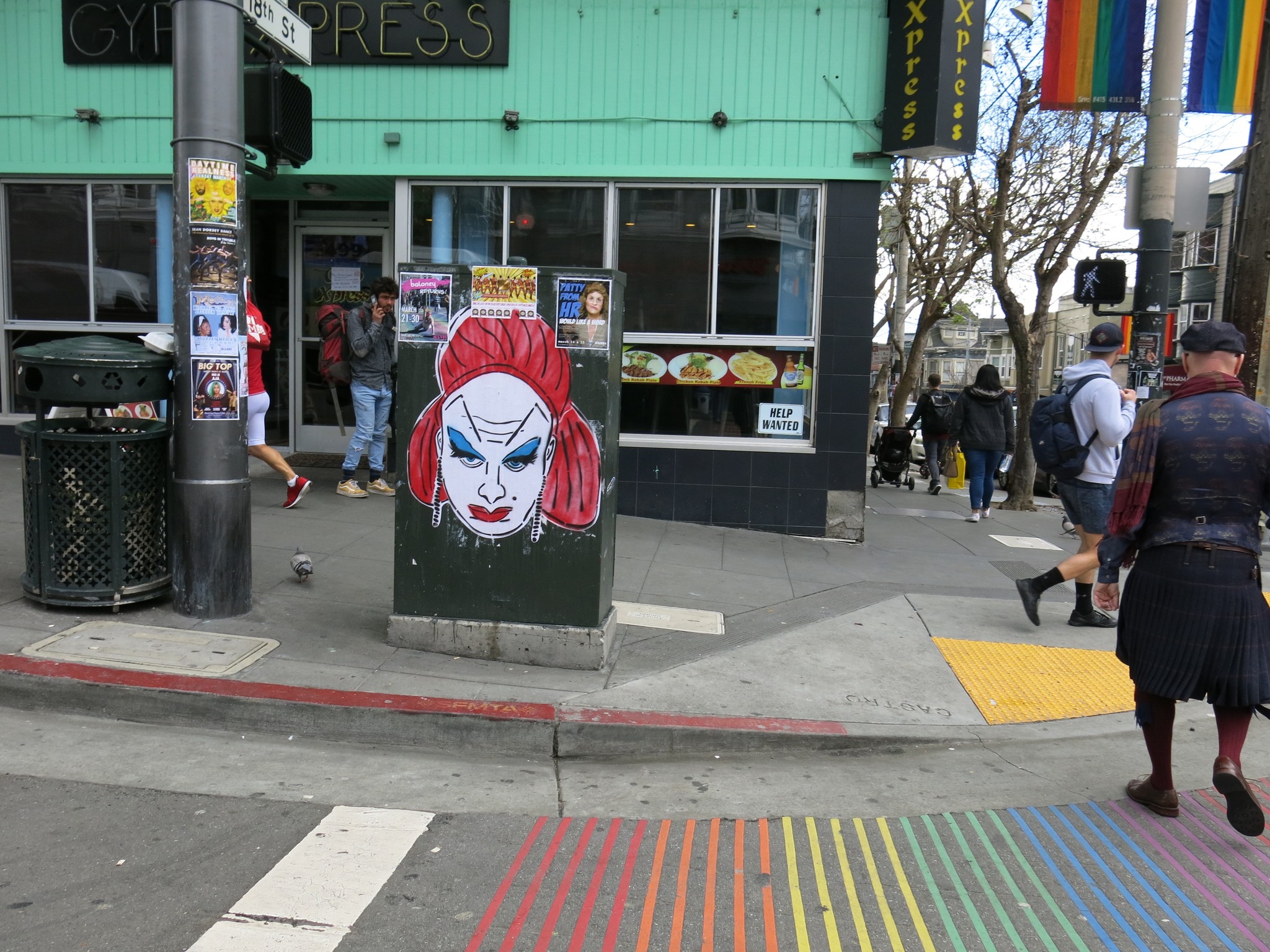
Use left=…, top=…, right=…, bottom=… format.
left=73, top=107, right=102, bottom=126
left=501, top=110, right=521, bottom=131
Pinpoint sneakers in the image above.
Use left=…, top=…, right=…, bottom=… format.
left=366, top=478, right=395, bottom=496
left=283, top=476, right=313, bottom=508
left=336, top=478, right=369, bottom=498
left=1069, top=609, right=1117, bottom=627
left=1015, top=577, right=1043, bottom=625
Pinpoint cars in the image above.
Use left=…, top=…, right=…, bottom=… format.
left=871, top=401, right=927, bottom=464
left=998, top=452, right=1058, bottom=496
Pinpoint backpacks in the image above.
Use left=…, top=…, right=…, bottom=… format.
left=1029, top=374, right=1119, bottom=479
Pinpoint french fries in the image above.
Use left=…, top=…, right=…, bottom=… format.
left=731, top=349, right=776, bottom=382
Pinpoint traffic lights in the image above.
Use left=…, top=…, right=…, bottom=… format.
left=244, top=66, right=316, bottom=168
left=1073, top=259, right=1127, bottom=307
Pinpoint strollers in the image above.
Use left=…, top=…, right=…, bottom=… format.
left=870, top=425, right=921, bottom=490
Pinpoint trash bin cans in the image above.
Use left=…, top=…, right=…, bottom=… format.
left=14, top=336, right=177, bottom=614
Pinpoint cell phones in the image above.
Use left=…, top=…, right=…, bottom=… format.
left=370, top=294, right=380, bottom=309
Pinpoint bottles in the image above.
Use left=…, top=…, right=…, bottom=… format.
left=796, top=354, right=805, bottom=384
left=999, top=454, right=1013, bottom=473
left=784, top=355, right=797, bottom=387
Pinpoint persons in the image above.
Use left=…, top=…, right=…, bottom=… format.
left=241, top=275, right=313, bottom=508
left=1019, top=323, right=1136, bottom=628
left=194, top=381, right=238, bottom=419
left=905, top=373, right=953, bottom=495
left=948, top=364, right=1017, bottom=522
left=217, top=314, right=236, bottom=337
left=403, top=290, right=450, bottom=336
left=336, top=276, right=399, bottom=498
left=189, top=243, right=238, bottom=284
left=194, top=181, right=233, bottom=214
left=1093, top=319, right=1270, bottom=837
left=193, top=316, right=212, bottom=336
left=560, top=280, right=610, bottom=348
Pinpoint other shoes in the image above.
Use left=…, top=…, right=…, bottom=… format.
left=966, top=513, right=980, bottom=522
left=927, top=485, right=942, bottom=495
left=980, top=500, right=990, bottom=518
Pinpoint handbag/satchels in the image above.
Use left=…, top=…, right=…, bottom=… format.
left=246, top=280, right=270, bottom=351
left=930, top=390, right=953, bottom=433
left=315, top=304, right=366, bottom=385
left=944, top=445, right=966, bottom=489
left=941, top=446, right=958, bottom=478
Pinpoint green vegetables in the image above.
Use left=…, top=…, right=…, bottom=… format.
left=690, top=353, right=715, bottom=369
left=624, top=351, right=658, bottom=369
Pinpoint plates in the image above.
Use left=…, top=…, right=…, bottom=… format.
left=621, top=350, right=667, bottom=379
left=113, top=404, right=153, bottom=419
left=668, top=354, right=728, bottom=380
left=728, top=352, right=778, bottom=381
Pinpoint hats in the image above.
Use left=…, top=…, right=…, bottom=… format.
left=1085, top=323, right=1124, bottom=353
left=1180, top=319, right=1247, bottom=356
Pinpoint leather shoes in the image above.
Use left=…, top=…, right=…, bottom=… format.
left=1213, top=755, right=1266, bottom=838
left=1126, top=773, right=1181, bottom=819
